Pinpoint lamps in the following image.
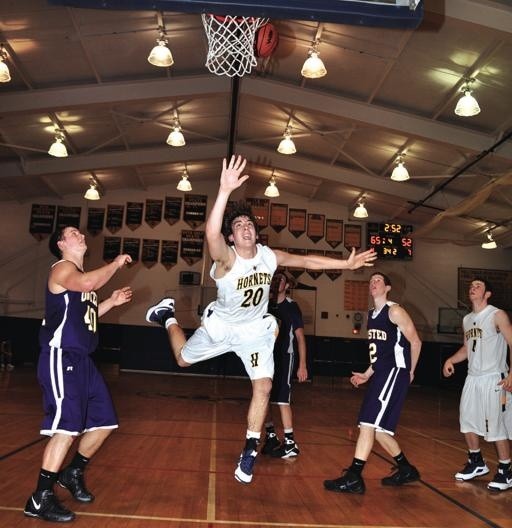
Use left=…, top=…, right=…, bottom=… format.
left=84, top=185, right=100, bottom=200
left=176, top=174, right=192, bottom=191
left=263, top=177, right=279, bottom=198
left=276, top=129, right=297, bottom=155
left=166, top=127, right=185, bottom=146
left=352, top=203, right=369, bottom=217
left=1, top=59, right=11, bottom=83
left=455, top=91, right=480, bottom=117
left=390, top=164, right=409, bottom=181
left=301, top=53, right=327, bottom=78
left=147, top=39, right=174, bottom=66
left=48, top=138, right=67, bottom=157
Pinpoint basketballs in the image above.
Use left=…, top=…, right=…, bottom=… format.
left=255, top=22, right=279, bottom=58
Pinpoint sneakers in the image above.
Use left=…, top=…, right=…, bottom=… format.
left=382, top=467, right=419, bottom=484
left=146, top=298, right=175, bottom=324
left=234, top=432, right=299, bottom=484
left=455, top=459, right=512, bottom=491
left=324, top=476, right=364, bottom=494
left=23, top=464, right=94, bottom=522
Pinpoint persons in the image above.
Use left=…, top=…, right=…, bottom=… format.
left=22, top=224, right=133, bottom=523
left=259, top=271, right=308, bottom=460
left=323, top=271, right=423, bottom=496
left=441, top=276, right=512, bottom=492
left=143, top=153, right=378, bottom=485
